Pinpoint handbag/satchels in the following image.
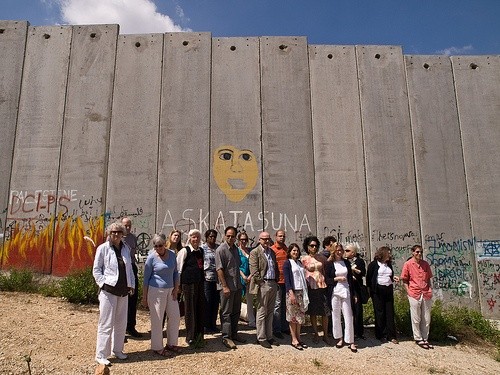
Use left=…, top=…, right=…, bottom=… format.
left=358, top=284, right=369, bottom=304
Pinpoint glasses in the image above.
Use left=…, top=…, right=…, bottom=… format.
left=261, top=238, right=272, bottom=241
left=111, top=230, right=123, bottom=235
left=416, top=250, right=423, bottom=254
left=345, top=250, right=350, bottom=252
left=227, top=235, right=235, bottom=238
left=154, top=244, right=163, bottom=248
left=309, top=244, right=318, bottom=248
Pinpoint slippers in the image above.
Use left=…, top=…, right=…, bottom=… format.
left=290, top=338, right=308, bottom=350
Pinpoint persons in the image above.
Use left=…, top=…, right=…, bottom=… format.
left=344, top=241, right=400, bottom=346
left=92, top=223, right=135, bottom=365
left=236, top=231, right=357, bottom=352
left=119, top=217, right=144, bottom=343
left=400, top=245, right=433, bottom=349
left=148, top=225, right=247, bottom=351
left=142, top=232, right=183, bottom=356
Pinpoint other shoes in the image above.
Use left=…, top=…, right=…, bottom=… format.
left=233, top=334, right=247, bottom=343
left=350, top=344, right=357, bottom=351
left=256, top=336, right=271, bottom=348
left=95, top=356, right=111, bottom=365
left=268, top=338, right=279, bottom=346
left=111, top=349, right=129, bottom=360
left=274, top=331, right=284, bottom=339
left=388, top=336, right=398, bottom=344
left=186, top=334, right=208, bottom=345
left=355, top=334, right=368, bottom=340
left=377, top=335, right=386, bottom=343
left=284, top=327, right=292, bottom=335
left=125, top=328, right=143, bottom=338
left=222, top=337, right=236, bottom=349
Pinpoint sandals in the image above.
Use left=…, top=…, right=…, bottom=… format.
left=323, top=335, right=333, bottom=346
left=313, top=334, right=319, bottom=343
left=155, top=347, right=173, bottom=357
left=166, top=344, right=183, bottom=353
left=416, top=340, right=433, bottom=349
left=335, top=339, right=344, bottom=348
